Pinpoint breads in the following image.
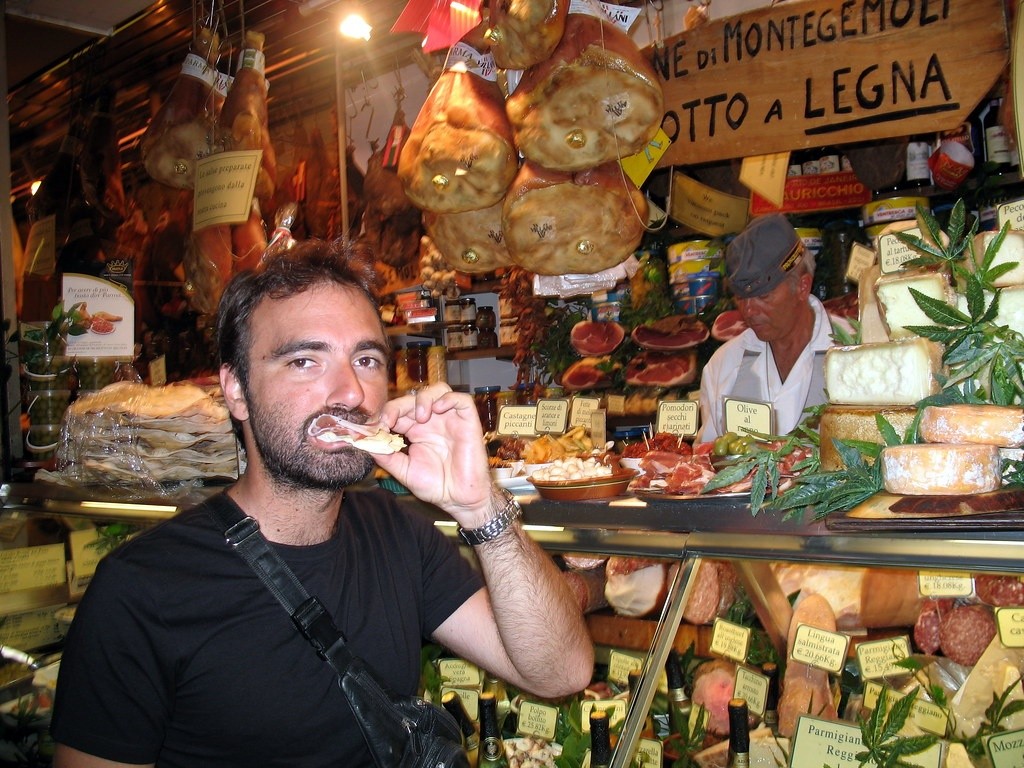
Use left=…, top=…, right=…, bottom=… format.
left=817, top=221, right=1024, bottom=494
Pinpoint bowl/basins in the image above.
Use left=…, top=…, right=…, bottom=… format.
left=619, top=457, right=647, bottom=476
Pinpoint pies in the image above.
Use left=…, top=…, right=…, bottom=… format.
left=307, top=414, right=406, bottom=455
left=66, top=380, right=240, bottom=482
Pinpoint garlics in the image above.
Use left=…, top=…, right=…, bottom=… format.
left=532, top=457, right=613, bottom=482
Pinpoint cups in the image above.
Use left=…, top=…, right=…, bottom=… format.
left=490, top=468, right=514, bottom=480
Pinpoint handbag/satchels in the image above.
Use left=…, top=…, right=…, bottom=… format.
left=336, top=657, right=471, bottom=768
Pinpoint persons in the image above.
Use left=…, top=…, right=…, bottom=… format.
left=13, top=193, right=56, bottom=323
left=47, top=237, right=595, bottom=767
left=691, top=212, right=858, bottom=445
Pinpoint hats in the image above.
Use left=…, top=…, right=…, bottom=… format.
left=721, top=212, right=805, bottom=298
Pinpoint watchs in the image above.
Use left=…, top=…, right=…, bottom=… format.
left=457, top=487, right=523, bottom=547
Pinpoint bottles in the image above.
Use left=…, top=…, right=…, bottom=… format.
left=589, top=711, right=610, bottom=768
left=787, top=98, right=1020, bottom=197
left=441, top=690, right=480, bottom=751
left=727, top=697, right=750, bottom=768
left=477, top=692, right=510, bottom=768
left=665, top=647, right=692, bottom=734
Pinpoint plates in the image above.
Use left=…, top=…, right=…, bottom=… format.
left=636, top=477, right=792, bottom=500
left=526, top=468, right=640, bottom=500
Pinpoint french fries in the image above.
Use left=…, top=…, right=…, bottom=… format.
left=524, top=426, right=595, bottom=465
